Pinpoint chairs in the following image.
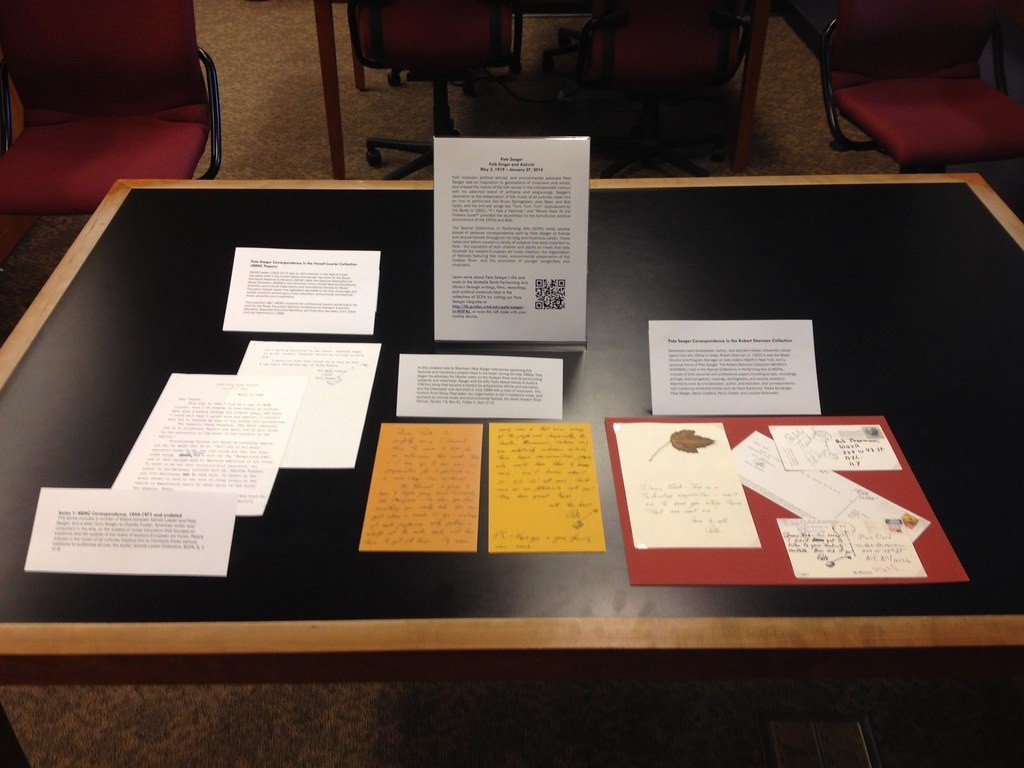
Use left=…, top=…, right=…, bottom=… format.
left=574, top=0, right=752, bottom=179
left=820, top=0, right=1024, bottom=173
left=0, top=0, right=221, bottom=216
left=348, top=0, right=525, bottom=180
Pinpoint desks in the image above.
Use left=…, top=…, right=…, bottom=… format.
left=0, top=172, right=1024, bottom=768
